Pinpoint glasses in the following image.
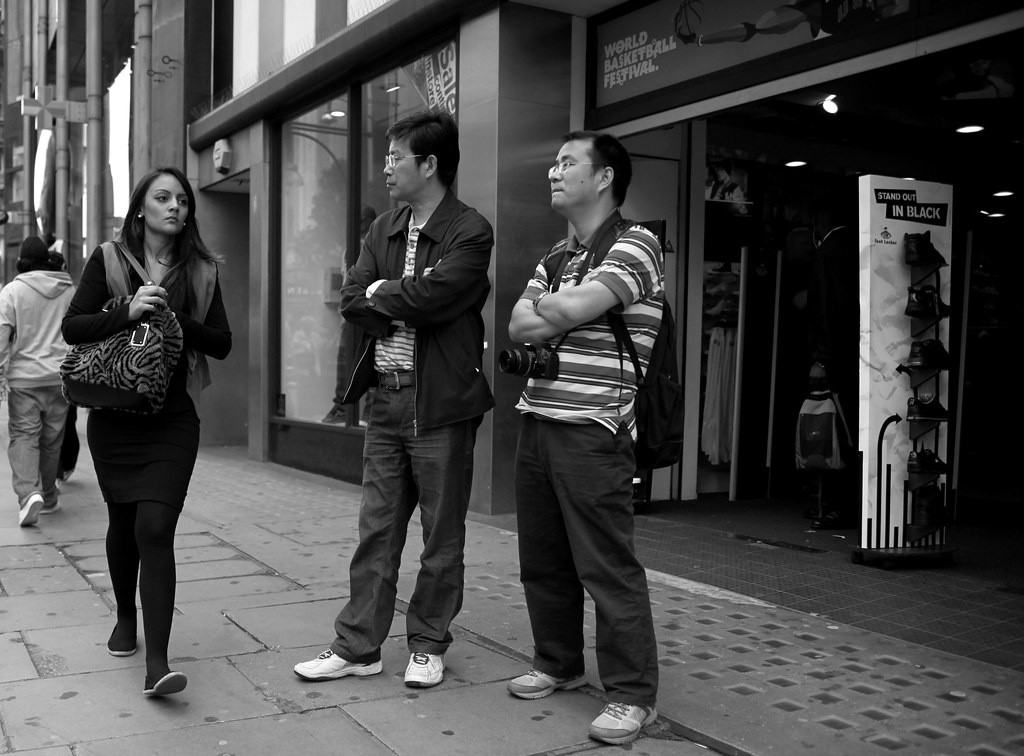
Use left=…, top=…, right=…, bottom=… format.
left=547, top=161, right=604, bottom=179
left=384, top=154, right=434, bottom=169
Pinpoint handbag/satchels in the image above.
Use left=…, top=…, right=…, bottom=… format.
left=59, top=240, right=187, bottom=414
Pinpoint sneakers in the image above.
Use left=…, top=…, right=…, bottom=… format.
left=588, top=695, right=658, bottom=745
left=293, top=646, right=383, bottom=681
left=403, top=651, right=444, bottom=686
left=507, top=665, right=589, bottom=700
left=901, top=231, right=951, bottom=537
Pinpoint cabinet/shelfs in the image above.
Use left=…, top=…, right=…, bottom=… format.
left=904, top=263, right=954, bottom=549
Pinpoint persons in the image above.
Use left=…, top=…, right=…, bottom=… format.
left=42, top=250, right=79, bottom=483
left=0, top=235, right=77, bottom=526
left=317, top=205, right=378, bottom=423
left=509, top=130, right=668, bottom=753
left=291, top=110, right=495, bottom=688
left=61, top=167, right=231, bottom=698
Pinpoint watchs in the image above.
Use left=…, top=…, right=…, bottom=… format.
left=532, top=293, right=551, bottom=315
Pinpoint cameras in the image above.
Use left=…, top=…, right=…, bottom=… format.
left=498, top=342, right=560, bottom=381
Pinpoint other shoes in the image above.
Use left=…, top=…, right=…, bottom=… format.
left=143, top=670, right=188, bottom=695
left=107, top=619, right=138, bottom=658
left=58, top=466, right=77, bottom=485
left=41, top=498, right=62, bottom=515
left=20, top=492, right=44, bottom=526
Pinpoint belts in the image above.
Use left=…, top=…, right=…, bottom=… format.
left=378, top=370, right=418, bottom=390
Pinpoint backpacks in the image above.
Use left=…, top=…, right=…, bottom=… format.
left=546, top=218, right=685, bottom=467
left=794, top=391, right=850, bottom=472
